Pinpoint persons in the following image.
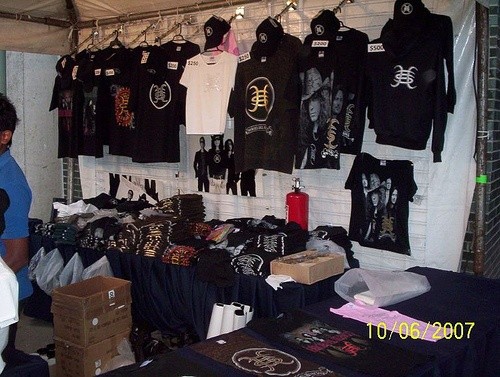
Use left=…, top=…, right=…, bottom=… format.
left=0, top=188, right=19, bottom=375
left=301, top=67, right=339, bottom=169
left=331, top=86, right=344, bottom=114
left=361, top=173, right=398, bottom=243
left=121, top=190, right=133, bottom=201
left=195, top=134, right=257, bottom=197
left=0, top=93, right=33, bottom=348
left=295, top=327, right=366, bottom=357
left=138, top=193, right=149, bottom=202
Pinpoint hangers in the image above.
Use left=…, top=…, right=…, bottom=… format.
left=109, top=29, right=124, bottom=49
left=332, top=6, right=351, bottom=30
left=138, top=30, right=149, bottom=48
left=172, top=23, right=188, bottom=42
left=153, top=36, right=166, bottom=50
left=202, top=46, right=223, bottom=54
left=87, top=34, right=98, bottom=50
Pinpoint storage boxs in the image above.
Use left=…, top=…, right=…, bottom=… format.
left=269, top=249, right=345, bottom=286
left=52, top=331, right=131, bottom=377
left=51, top=275, right=132, bottom=347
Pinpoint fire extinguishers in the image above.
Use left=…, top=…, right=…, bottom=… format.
left=285, top=176, right=309, bottom=231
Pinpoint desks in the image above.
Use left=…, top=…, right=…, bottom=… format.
left=90, top=267, right=500, bottom=377
left=20, top=234, right=359, bottom=341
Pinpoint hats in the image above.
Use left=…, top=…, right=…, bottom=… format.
left=253, top=16, right=285, bottom=58
left=203, top=15, right=231, bottom=49
left=309, top=8, right=340, bottom=58
